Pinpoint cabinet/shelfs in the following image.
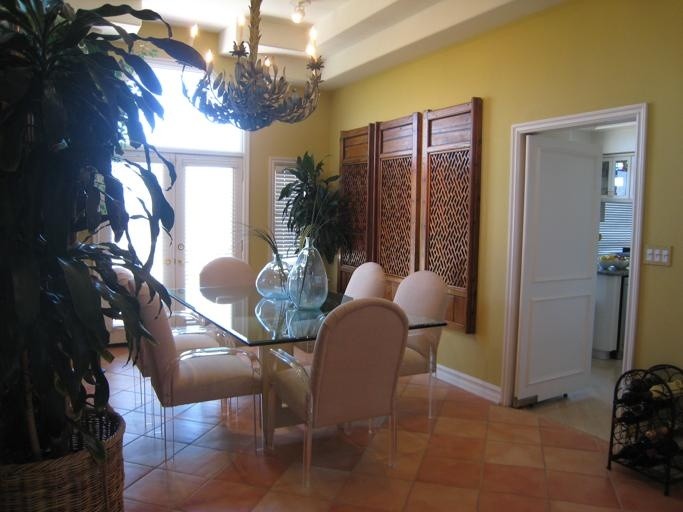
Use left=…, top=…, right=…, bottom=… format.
left=601, top=152, right=635, bottom=203
left=607, top=363, right=683, bottom=496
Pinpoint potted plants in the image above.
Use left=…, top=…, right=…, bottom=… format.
left=0, top=0, right=205, bottom=512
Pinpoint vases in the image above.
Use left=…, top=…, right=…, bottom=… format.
left=256, top=254, right=293, bottom=300
left=286, top=309, right=325, bottom=339
left=255, top=297, right=297, bottom=339
left=287, top=236, right=329, bottom=310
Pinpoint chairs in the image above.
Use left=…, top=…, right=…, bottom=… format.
left=342, top=261, right=449, bottom=437
left=112, top=257, right=262, bottom=465
left=268, top=297, right=407, bottom=488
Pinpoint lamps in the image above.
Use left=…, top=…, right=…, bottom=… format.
left=189, top=0, right=317, bottom=68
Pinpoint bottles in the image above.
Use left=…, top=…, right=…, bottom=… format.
left=614, top=373, right=682, bottom=469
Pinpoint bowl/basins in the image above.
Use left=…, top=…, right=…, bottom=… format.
left=597, top=255, right=629, bottom=270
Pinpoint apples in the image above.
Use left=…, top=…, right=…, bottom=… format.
left=601, top=255, right=627, bottom=261
